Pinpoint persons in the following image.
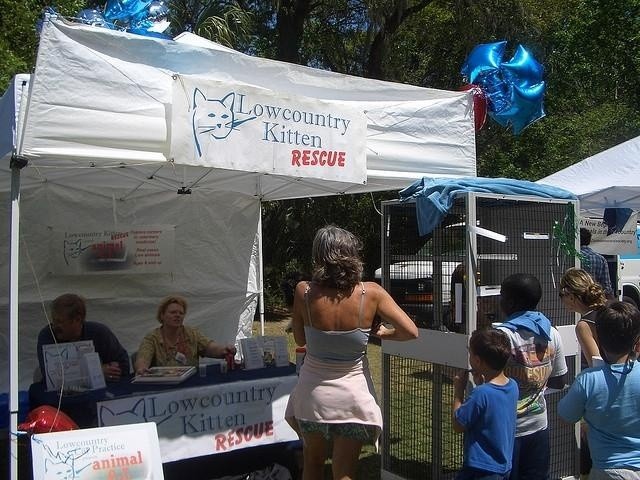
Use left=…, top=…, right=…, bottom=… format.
left=557, top=266, right=640, bottom=480
left=284, top=223, right=420, bottom=480
left=491, top=272, right=569, bottom=480
left=580, top=227, right=616, bottom=300
left=556, top=300, right=640, bottom=480
left=28, top=293, right=131, bottom=429
left=450, top=325, right=520, bottom=480
left=132, top=296, right=237, bottom=377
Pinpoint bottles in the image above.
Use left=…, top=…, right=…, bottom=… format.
left=295, top=347, right=306, bottom=376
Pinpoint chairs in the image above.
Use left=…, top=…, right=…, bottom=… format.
left=131, top=350, right=156, bottom=371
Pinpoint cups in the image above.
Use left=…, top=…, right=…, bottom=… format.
left=198, top=361, right=207, bottom=378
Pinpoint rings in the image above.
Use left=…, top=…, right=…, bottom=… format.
left=109, top=375, right=113, bottom=379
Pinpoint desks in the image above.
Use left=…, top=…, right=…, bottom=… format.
left=0, top=360, right=302, bottom=480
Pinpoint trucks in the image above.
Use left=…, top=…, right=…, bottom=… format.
left=375, top=220, right=640, bottom=313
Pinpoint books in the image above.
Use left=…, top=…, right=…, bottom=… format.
left=131, top=365, right=197, bottom=386
